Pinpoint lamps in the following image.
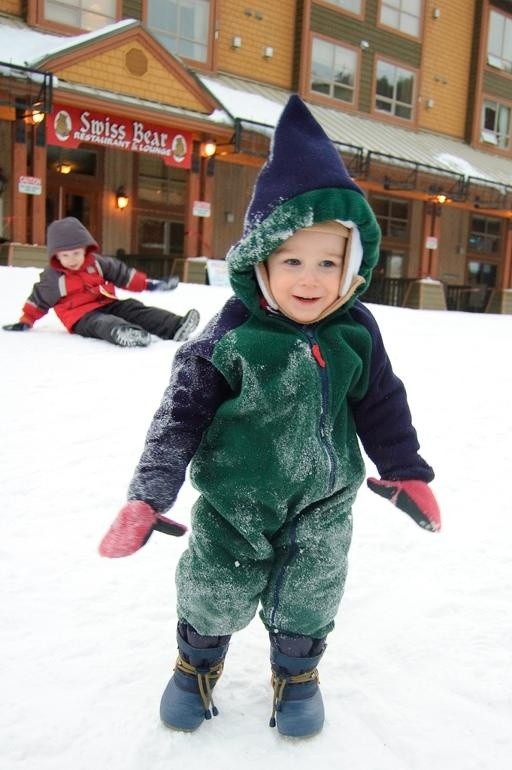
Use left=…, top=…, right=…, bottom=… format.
left=434, top=184, right=446, bottom=205
left=116, top=184, right=129, bottom=212
left=204, top=133, right=218, bottom=159
left=53, top=159, right=75, bottom=179
left=27, top=103, right=50, bottom=125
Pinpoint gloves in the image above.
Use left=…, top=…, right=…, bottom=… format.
left=2, top=322, right=30, bottom=332
left=366, top=476, right=441, bottom=533
left=98, top=500, right=188, bottom=559
left=147, top=275, right=179, bottom=292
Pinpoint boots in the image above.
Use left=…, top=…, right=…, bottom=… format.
left=268, top=631, right=328, bottom=739
left=159, top=622, right=233, bottom=733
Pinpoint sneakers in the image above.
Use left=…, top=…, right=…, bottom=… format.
left=110, top=325, right=151, bottom=348
left=170, top=309, right=200, bottom=342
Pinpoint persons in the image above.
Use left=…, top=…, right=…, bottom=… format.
left=98, top=95, right=442, bottom=741
left=2, top=217, right=201, bottom=348
